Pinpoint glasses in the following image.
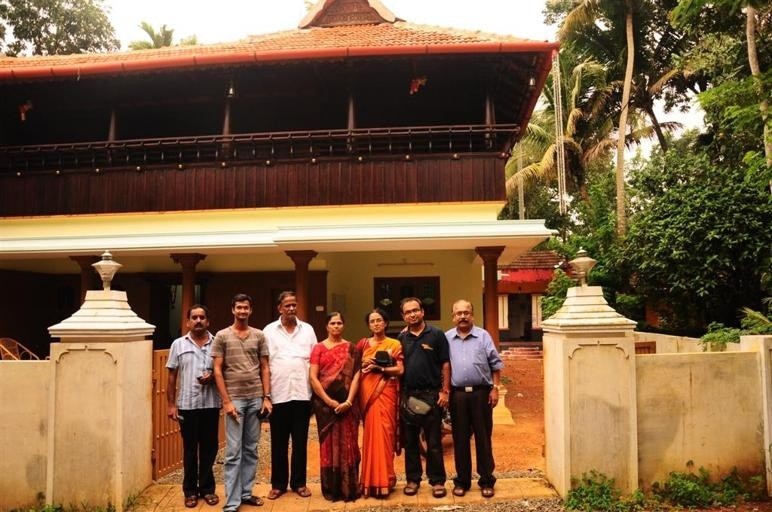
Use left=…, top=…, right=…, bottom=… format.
left=403, top=307, right=421, bottom=315
left=453, top=311, right=472, bottom=317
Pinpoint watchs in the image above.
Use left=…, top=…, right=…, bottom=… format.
left=262, top=393, right=272, bottom=399
left=344, top=399, right=353, bottom=407
left=493, top=384, right=501, bottom=391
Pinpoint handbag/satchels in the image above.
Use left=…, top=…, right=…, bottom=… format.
left=400, top=391, right=441, bottom=418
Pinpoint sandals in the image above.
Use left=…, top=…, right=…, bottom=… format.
left=203, top=493, right=219, bottom=506
left=453, top=485, right=468, bottom=497
left=292, top=487, right=311, bottom=497
left=241, top=495, right=264, bottom=507
left=185, top=495, right=198, bottom=508
left=432, top=485, right=447, bottom=498
left=404, top=481, right=419, bottom=496
left=481, top=486, right=494, bottom=497
left=267, top=488, right=284, bottom=500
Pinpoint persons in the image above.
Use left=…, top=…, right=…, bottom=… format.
left=263, top=290, right=319, bottom=500
left=310, top=309, right=360, bottom=503
left=165, top=303, right=223, bottom=507
left=211, top=293, right=274, bottom=511
left=355, top=307, right=405, bottom=499
left=396, top=296, right=452, bottom=498
left=442, top=298, right=505, bottom=498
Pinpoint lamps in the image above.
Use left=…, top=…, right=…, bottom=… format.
left=563, top=242, right=599, bottom=289
left=90, top=248, right=125, bottom=293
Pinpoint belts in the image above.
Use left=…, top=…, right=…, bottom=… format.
left=451, top=384, right=489, bottom=393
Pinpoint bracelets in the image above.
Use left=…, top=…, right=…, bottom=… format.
left=440, top=389, right=450, bottom=394
